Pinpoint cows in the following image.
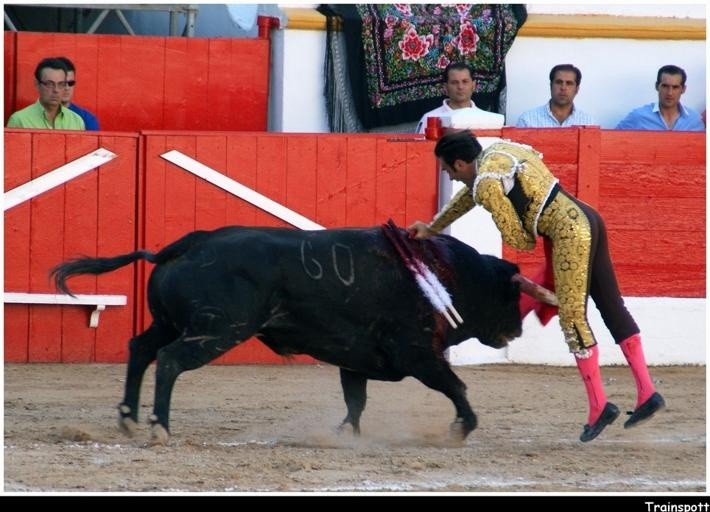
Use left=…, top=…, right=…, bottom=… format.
left=48, top=226, right=559, bottom=448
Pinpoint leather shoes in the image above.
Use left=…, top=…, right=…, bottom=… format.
left=623, top=392, right=667, bottom=429
left=579, top=402, right=621, bottom=442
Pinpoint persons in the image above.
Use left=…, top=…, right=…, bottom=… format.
left=55, top=56, right=99, bottom=131
left=408, top=130, right=666, bottom=441
left=5, top=58, right=86, bottom=131
left=517, top=65, right=596, bottom=128
left=417, top=62, right=505, bottom=134
left=615, top=65, right=705, bottom=132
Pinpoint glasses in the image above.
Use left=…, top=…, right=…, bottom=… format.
left=39, top=80, right=76, bottom=89
